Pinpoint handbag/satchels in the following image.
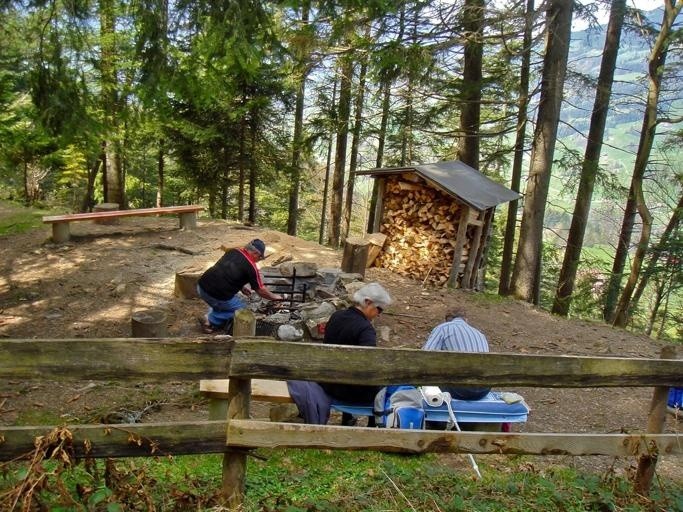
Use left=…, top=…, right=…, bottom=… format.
left=374, top=383, right=426, bottom=430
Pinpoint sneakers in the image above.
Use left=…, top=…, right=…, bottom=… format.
left=198, top=315, right=213, bottom=334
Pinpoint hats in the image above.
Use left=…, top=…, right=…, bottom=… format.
left=250, top=238, right=265, bottom=260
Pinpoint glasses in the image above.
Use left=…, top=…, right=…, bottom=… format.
left=376, top=304, right=384, bottom=314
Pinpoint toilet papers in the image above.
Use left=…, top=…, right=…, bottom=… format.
left=420, top=385, right=445, bottom=408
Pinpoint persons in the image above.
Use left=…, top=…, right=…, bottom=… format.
left=318, top=283, right=391, bottom=426
left=194, top=239, right=284, bottom=334
left=420, top=307, right=492, bottom=400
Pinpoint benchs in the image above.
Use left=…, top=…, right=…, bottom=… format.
left=198, top=378, right=529, bottom=431
left=41, top=203, right=205, bottom=244
